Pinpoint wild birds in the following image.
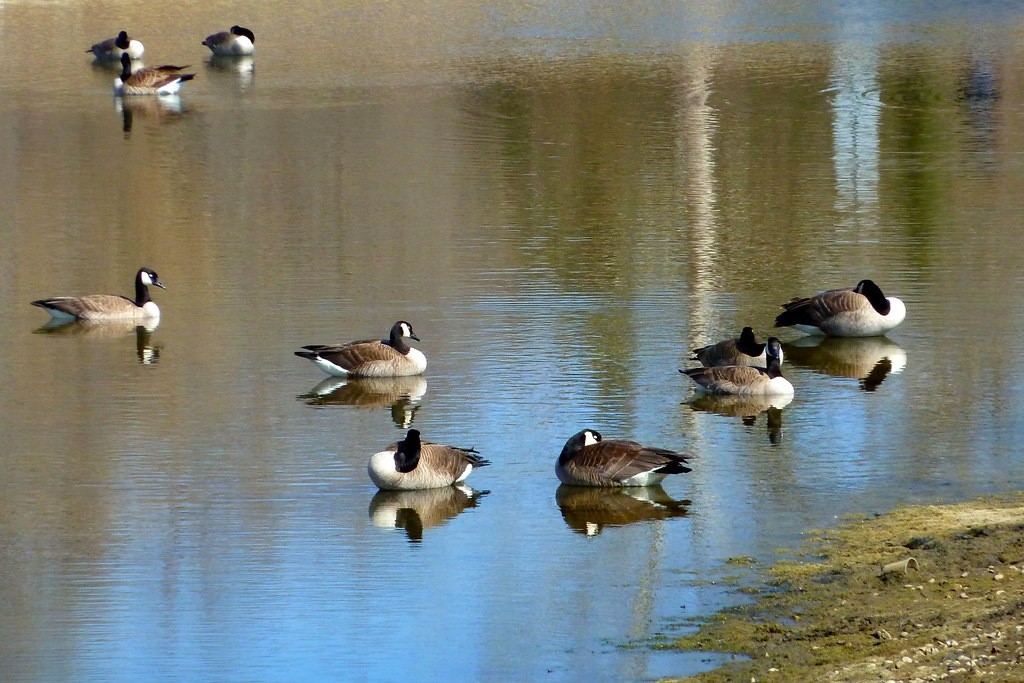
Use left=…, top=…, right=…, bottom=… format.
left=84, top=30, right=146, bottom=60
left=773, top=278, right=906, bottom=338
left=201, top=24, right=256, bottom=56
left=113, top=52, right=198, bottom=93
left=367, top=429, right=493, bottom=492
left=293, top=320, right=427, bottom=377
left=364, top=484, right=490, bottom=540
left=688, top=327, right=784, bottom=369
left=291, top=377, right=427, bottom=428
left=677, top=337, right=795, bottom=396
left=30, top=267, right=167, bottom=320
left=783, top=340, right=909, bottom=394
left=114, top=93, right=185, bottom=138
left=554, top=428, right=694, bottom=487
left=32, top=323, right=163, bottom=368
left=681, top=396, right=794, bottom=446
left=556, top=488, right=695, bottom=539
